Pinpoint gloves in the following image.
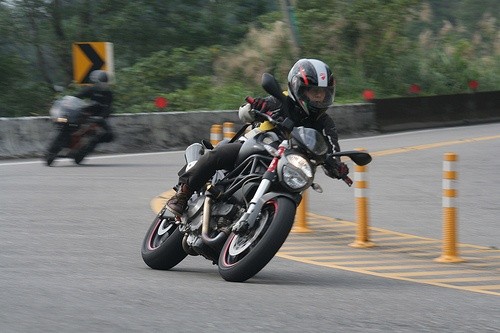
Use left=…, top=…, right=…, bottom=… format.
left=334, top=161, right=350, bottom=180
left=251, top=97, right=274, bottom=117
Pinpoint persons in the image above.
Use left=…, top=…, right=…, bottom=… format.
left=164, top=58, right=350, bottom=217
left=71, top=69, right=116, bottom=151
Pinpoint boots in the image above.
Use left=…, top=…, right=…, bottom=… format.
left=166, top=183, right=193, bottom=215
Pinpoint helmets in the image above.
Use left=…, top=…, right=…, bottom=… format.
left=91, top=69, right=112, bottom=83
left=287, top=58, right=335, bottom=118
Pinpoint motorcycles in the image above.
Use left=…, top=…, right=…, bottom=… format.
left=141, top=73, right=373, bottom=283
left=43, top=84, right=117, bottom=167
